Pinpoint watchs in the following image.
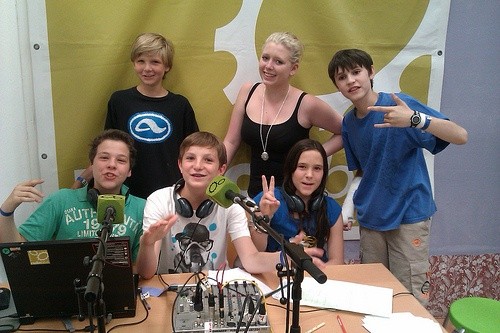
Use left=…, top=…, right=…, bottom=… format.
left=75, top=176, right=87, bottom=186
left=410, top=110, right=421, bottom=128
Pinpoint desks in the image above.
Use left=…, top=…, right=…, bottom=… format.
left=0, top=262, right=449, bottom=333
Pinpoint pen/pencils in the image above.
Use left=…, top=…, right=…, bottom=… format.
left=307, top=322, right=326, bottom=333
left=337, top=316, right=346, bottom=333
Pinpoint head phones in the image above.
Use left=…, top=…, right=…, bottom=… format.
left=86, top=178, right=129, bottom=210
left=282, top=177, right=326, bottom=213
left=172, top=177, right=214, bottom=218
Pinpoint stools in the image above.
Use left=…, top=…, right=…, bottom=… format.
left=450, top=296, right=500, bottom=333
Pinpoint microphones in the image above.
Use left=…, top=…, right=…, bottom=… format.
left=204, top=176, right=241, bottom=208
left=98, top=193, right=126, bottom=226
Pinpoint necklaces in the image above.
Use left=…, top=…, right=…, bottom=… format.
left=261, top=84, right=290, bottom=160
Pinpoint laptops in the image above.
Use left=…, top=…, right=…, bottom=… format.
left=0, top=235, right=140, bottom=324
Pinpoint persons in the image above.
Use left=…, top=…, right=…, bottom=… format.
left=221, top=30, right=344, bottom=201
left=137, top=131, right=301, bottom=279
left=241, top=137, right=343, bottom=267
left=0, top=129, right=148, bottom=267
left=72, top=31, right=200, bottom=203
left=328, top=50, right=468, bottom=309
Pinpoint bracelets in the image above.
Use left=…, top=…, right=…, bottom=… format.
left=0, top=208, right=14, bottom=216
left=279, top=252, right=284, bottom=265
left=251, top=223, right=265, bottom=233
left=422, top=115, right=432, bottom=130
